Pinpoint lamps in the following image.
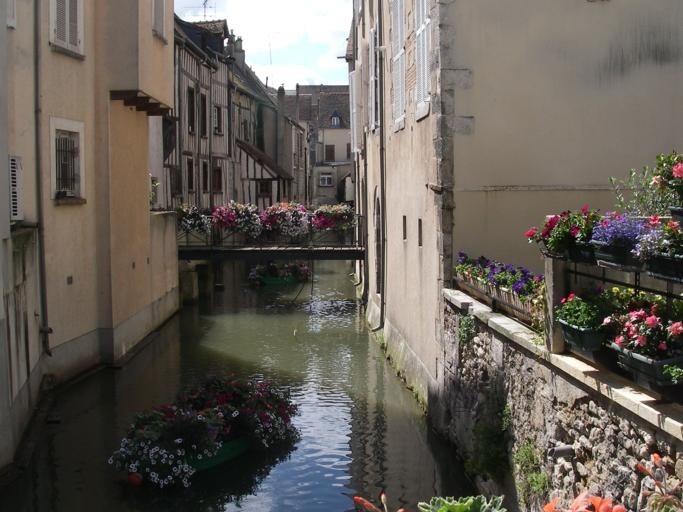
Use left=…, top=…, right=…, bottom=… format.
left=330, top=110, right=350, bottom=127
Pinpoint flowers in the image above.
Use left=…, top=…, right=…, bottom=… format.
left=555, top=289, right=606, bottom=326
left=108, top=375, right=303, bottom=492
left=456, top=249, right=544, bottom=295
left=247, top=262, right=312, bottom=289
left=609, top=285, right=682, bottom=360
left=522, top=149, right=681, bottom=276
left=175, top=199, right=356, bottom=241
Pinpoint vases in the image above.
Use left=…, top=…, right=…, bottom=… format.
left=557, top=320, right=615, bottom=359
left=222, top=225, right=246, bottom=247
left=177, top=227, right=212, bottom=246
left=312, top=228, right=340, bottom=247
left=605, top=336, right=676, bottom=384
